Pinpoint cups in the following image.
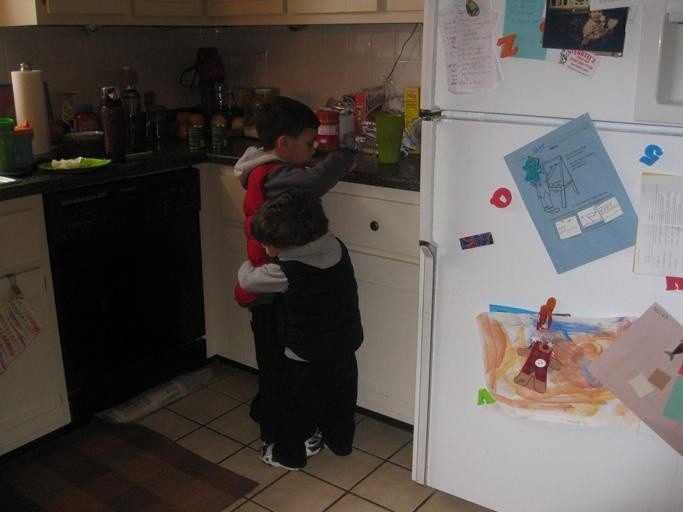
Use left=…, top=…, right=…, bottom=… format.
left=373, top=110, right=405, bottom=166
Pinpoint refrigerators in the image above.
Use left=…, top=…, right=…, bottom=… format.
left=410, top=1, right=683, bottom=512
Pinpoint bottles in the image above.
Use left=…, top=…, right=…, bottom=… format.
left=0, top=116, right=35, bottom=175
left=69, top=82, right=171, bottom=153
left=247, top=87, right=275, bottom=113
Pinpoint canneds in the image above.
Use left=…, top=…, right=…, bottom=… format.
left=315, top=107, right=340, bottom=148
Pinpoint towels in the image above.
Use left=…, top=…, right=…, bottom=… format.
left=0, top=297, right=40, bottom=374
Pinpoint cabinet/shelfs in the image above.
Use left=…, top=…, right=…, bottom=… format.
left=212, top=162, right=259, bottom=377
left=0, top=189, right=79, bottom=464
left=320, top=181, right=421, bottom=432
left=0, top=0, right=424, bottom=29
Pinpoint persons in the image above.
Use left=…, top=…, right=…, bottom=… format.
left=581, top=4, right=625, bottom=53
left=234, top=94, right=356, bottom=458
left=237, top=189, right=364, bottom=470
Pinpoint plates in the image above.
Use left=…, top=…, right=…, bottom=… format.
left=37, top=156, right=112, bottom=175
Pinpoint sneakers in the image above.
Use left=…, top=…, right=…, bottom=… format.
left=259, top=428, right=323, bottom=471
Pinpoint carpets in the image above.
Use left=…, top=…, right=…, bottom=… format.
left=0, top=422, right=260, bottom=512
